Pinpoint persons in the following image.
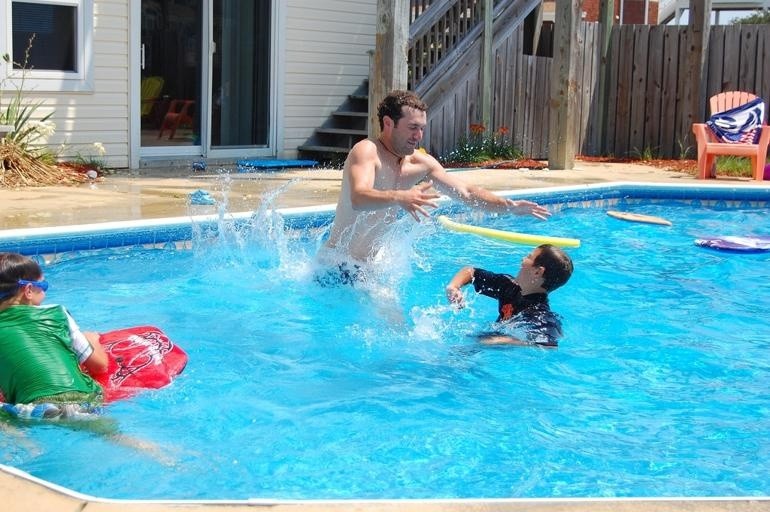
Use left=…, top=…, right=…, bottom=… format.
left=315, top=90, right=552, bottom=288
left=445, top=243, right=574, bottom=350
left=0, top=252, right=176, bottom=469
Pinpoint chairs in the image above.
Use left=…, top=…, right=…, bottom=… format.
left=141, top=77, right=198, bottom=142
left=692, top=91, right=770, bottom=181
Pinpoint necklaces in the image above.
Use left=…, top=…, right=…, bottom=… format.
left=378, top=137, right=405, bottom=165
